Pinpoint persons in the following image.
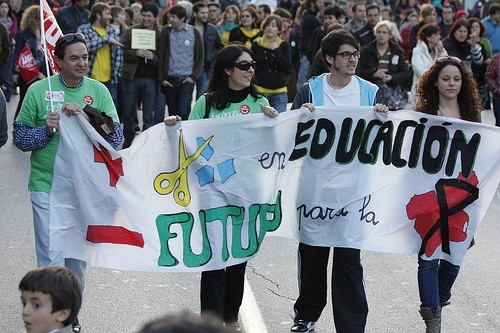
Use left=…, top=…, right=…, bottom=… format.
left=0, top=0, right=53, bottom=149
left=18, top=266, right=83, bottom=333
left=343, top=0, right=500, bottom=127
left=413, top=56, right=482, bottom=333
left=289, top=29, right=389, bottom=333
left=216, top=0, right=355, bottom=113
left=164, top=43, right=277, bottom=333
left=53, top=0, right=225, bottom=150
left=12, top=34, right=124, bottom=333
left=136, top=310, right=243, bottom=333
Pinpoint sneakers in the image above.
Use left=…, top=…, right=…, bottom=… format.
left=291, top=319, right=315, bottom=333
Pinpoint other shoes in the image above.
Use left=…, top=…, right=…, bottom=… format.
left=226, top=322, right=242, bottom=333
left=122, top=139, right=132, bottom=149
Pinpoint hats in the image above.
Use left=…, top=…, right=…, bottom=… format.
left=141, top=4, right=159, bottom=18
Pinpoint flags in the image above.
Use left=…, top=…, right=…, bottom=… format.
left=42, top=0, right=65, bottom=75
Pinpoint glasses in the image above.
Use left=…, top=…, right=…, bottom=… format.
left=436, top=57, right=461, bottom=64
left=242, top=14, right=251, bottom=18
left=232, top=61, right=256, bottom=71
left=336, top=51, right=361, bottom=59
left=56, top=33, right=84, bottom=42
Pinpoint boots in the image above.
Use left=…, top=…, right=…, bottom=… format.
left=419, top=304, right=442, bottom=333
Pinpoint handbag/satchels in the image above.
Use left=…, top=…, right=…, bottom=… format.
left=376, top=85, right=406, bottom=111
